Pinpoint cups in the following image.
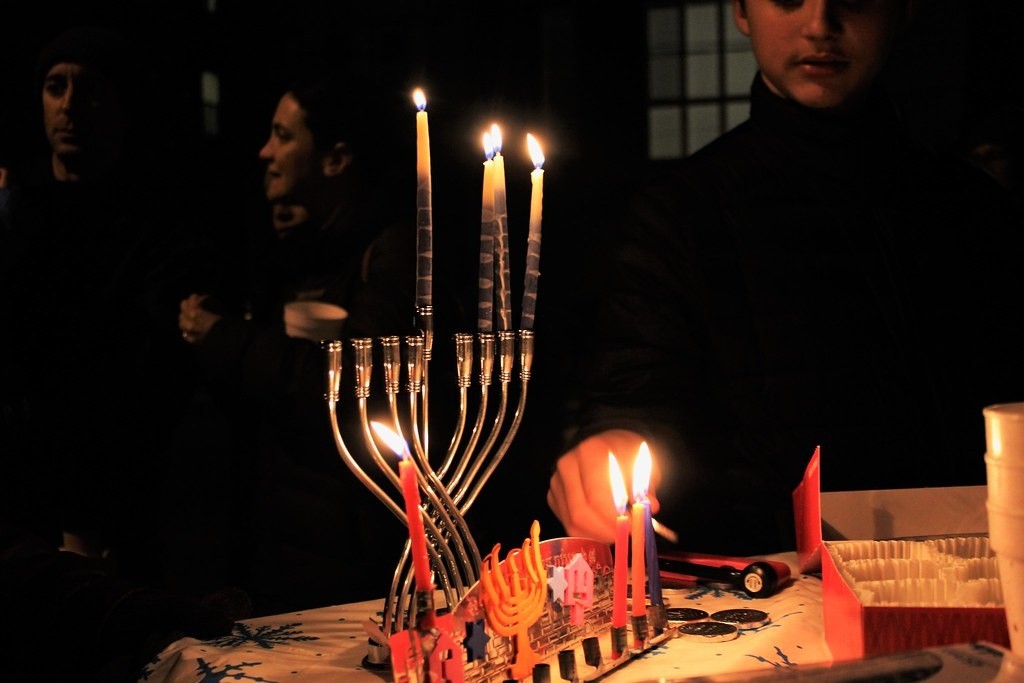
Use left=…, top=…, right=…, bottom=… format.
left=983, top=403, right=1024, bottom=660
left=286, top=301, right=350, bottom=340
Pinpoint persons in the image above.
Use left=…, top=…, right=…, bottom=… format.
left=179, top=86, right=416, bottom=610
left=0, top=25, right=221, bottom=581
left=546, top=0, right=1024, bottom=544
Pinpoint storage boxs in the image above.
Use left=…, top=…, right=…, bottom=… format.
left=791, top=441, right=1008, bottom=662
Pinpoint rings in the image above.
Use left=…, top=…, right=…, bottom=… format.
left=182, top=333, right=187, bottom=339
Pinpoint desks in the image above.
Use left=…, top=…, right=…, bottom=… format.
left=139, top=549, right=1024, bottom=683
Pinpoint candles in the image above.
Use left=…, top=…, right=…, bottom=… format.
left=603, top=441, right=662, bottom=627
left=476, top=124, right=546, bottom=332
left=412, top=88, right=432, bottom=308
left=371, top=421, right=434, bottom=592
